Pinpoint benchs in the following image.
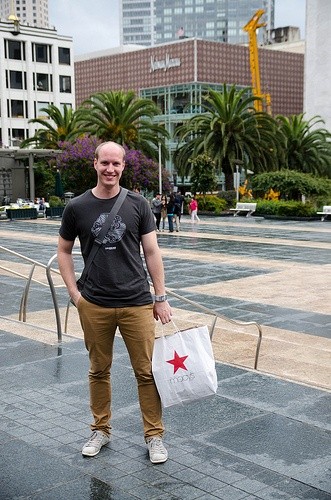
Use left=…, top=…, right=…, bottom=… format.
left=229, top=202, right=258, bottom=218
left=316, top=205, right=331, bottom=222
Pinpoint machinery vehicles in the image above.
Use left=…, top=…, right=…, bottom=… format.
left=235, top=7, right=282, bottom=202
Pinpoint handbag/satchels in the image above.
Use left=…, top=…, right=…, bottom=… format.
left=69, top=279, right=84, bottom=307
left=152, top=320, right=218, bottom=408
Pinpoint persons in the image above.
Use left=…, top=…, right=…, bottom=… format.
left=17, top=197, right=48, bottom=218
left=57, top=141, right=173, bottom=463
left=133, top=185, right=200, bottom=232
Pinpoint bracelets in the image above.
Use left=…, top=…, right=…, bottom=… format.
left=155, top=294, right=167, bottom=302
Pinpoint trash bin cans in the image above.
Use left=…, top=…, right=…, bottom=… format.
left=65, top=192, right=74, bottom=204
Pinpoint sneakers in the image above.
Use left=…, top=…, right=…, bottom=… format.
left=146, top=437, right=168, bottom=464
left=81, top=430, right=110, bottom=456
